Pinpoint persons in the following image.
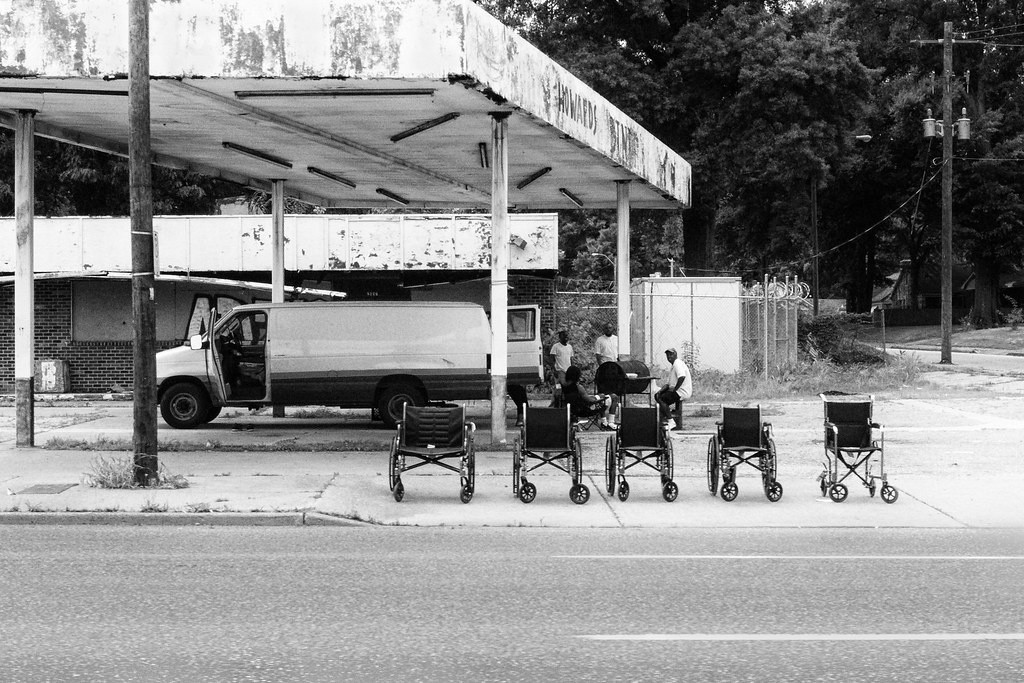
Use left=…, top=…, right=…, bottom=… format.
left=594, top=323, right=620, bottom=365
left=653, top=347, right=693, bottom=430
left=507, top=384, right=529, bottom=427
left=565, top=366, right=620, bottom=431
left=549, top=331, right=574, bottom=407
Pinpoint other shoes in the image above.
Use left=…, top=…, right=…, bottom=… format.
left=601, top=422, right=620, bottom=431
left=515, top=420, right=524, bottom=427
left=663, top=422, right=676, bottom=430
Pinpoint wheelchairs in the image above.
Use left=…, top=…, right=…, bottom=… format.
left=705, top=403, right=784, bottom=502
left=603, top=401, right=680, bottom=502
left=819, top=392, right=898, bottom=504
left=387, top=401, right=477, bottom=504
left=511, top=402, right=590, bottom=504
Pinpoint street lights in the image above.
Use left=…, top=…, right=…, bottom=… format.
left=591, top=252, right=617, bottom=307
left=811, top=134, right=872, bottom=317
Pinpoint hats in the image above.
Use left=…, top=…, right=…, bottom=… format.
left=665, top=347, right=676, bottom=353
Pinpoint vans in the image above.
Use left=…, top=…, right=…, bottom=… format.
left=156, top=299, right=547, bottom=430
